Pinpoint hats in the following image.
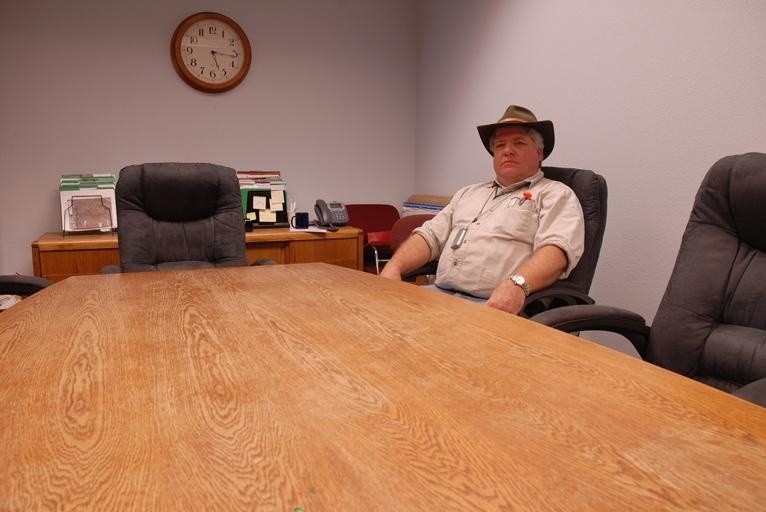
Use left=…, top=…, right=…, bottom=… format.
left=476, top=105, right=554, bottom=160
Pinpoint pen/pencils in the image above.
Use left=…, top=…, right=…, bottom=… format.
left=289, top=198, right=297, bottom=212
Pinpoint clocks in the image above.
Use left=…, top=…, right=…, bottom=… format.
left=171, top=11, right=252, bottom=93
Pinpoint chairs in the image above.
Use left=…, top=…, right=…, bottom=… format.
left=0, top=274, right=52, bottom=313
left=527, top=152, right=765, bottom=407
left=96, top=162, right=277, bottom=274
left=400, top=166, right=607, bottom=337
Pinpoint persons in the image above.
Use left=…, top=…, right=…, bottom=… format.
left=379, top=104, right=586, bottom=316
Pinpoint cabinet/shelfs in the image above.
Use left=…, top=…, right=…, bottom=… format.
left=32, top=221, right=364, bottom=282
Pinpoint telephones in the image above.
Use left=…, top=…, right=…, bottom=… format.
left=314, top=200, right=349, bottom=231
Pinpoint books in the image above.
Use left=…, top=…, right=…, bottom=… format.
left=404, top=203, right=443, bottom=211
left=407, top=193, right=453, bottom=207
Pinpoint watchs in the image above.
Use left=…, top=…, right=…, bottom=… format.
left=507, top=273, right=530, bottom=296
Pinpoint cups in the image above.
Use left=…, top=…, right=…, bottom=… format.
left=291, top=212, right=309, bottom=229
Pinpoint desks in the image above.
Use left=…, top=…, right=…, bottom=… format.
left=0, top=257, right=766, bottom=512
